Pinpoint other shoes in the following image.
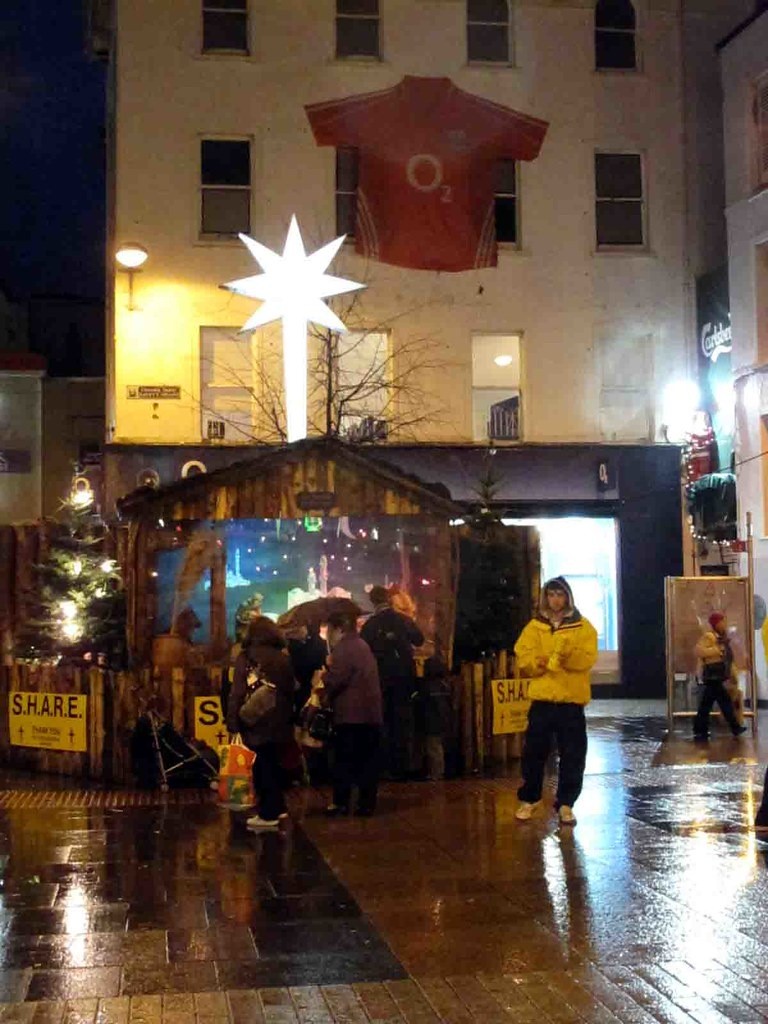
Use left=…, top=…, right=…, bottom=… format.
left=516, top=803, right=537, bottom=820
left=279, top=813, right=286, bottom=819
left=559, top=805, right=574, bottom=825
left=696, top=731, right=710, bottom=738
left=247, top=816, right=279, bottom=826
left=323, top=806, right=349, bottom=816
left=355, top=807, right=374, bottom=819
left=733, top=727, right=747, bottom=736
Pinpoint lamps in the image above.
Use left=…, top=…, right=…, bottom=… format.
left=115, top=239, right=149, bottom=313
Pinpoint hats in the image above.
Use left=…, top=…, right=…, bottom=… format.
left=709, top=613, right=723, bottom=625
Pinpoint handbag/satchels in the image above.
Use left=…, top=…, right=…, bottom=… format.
left=218, top=733, right=258, bottom=812
left=309, top=699, right=337, bottom=741
left=702, top=661, right=730, bottom=682
left=237, top=650, right=278, bottom=729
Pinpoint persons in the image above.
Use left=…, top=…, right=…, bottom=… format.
left=692, top=611, right=747, bottom=742
left=515, top=574, right=597, bottom=824
left=754, top=612, right=768, bottom=826
left=227, top=584, right=429, bottom=825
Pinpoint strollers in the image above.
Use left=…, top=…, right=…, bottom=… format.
left=127, top=681, right=221, bottom=795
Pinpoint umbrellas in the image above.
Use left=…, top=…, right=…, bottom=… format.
left=278, top=594, right=375, bottom=653
